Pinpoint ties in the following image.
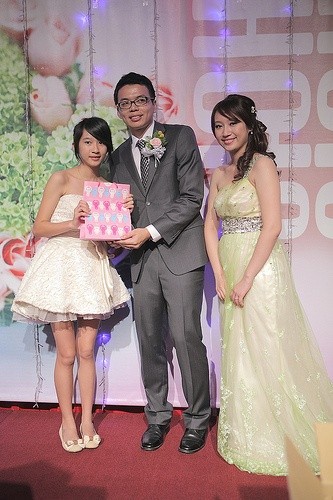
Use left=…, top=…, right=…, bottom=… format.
left=136, top=139, right=150, bottom=190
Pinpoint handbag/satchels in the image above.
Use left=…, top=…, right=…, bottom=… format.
left=79, top=181, right=131, bottom=241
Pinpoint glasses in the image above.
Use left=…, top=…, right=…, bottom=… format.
left=117, top=96, right=154, bottom=109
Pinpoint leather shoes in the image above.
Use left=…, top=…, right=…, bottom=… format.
left=141, top=424, right=170, bottom=451
left=179, top=428, right=208, bottom=454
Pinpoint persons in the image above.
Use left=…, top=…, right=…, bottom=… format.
left=10, top=117, right=133, bottom=452
left=106, top=72, right=217, bottom=454
left=203, top=94, right=333, bottom=476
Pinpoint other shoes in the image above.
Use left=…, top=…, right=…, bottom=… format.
left=58, top=423, right=85, bottom=452
left=80, top=423, right=101, bottom=449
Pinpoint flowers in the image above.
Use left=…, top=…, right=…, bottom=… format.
left=141, top=130, right=168, bottom=164
